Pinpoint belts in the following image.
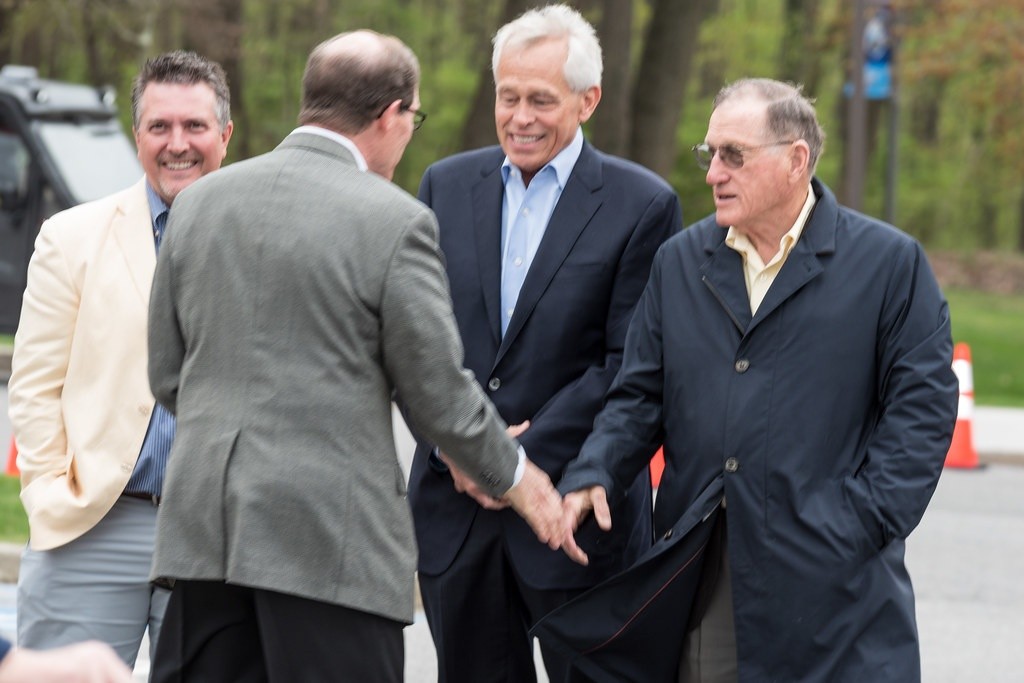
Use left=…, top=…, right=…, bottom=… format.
left=120, top=490, right=165, bottom=507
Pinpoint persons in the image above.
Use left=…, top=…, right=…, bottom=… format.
left=0, top=638, right=135, bottom=683
left=8, top=49, right=234, bottom=682
left=147, top=29, right=566, bottom=683
left=407, top=4, right=679, bottom=683
left=558, top=78, right=959, bottom=683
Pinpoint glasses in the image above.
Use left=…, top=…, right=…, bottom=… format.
left=693, top=137, right=799, bottom=173
left=399, top=106, right=427, bottom=129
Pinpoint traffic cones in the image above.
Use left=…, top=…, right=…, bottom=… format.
left=941, top=339, right=986, bottom=472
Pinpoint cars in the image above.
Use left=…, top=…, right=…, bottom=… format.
left=0, top=68, right=161, bottom=346
